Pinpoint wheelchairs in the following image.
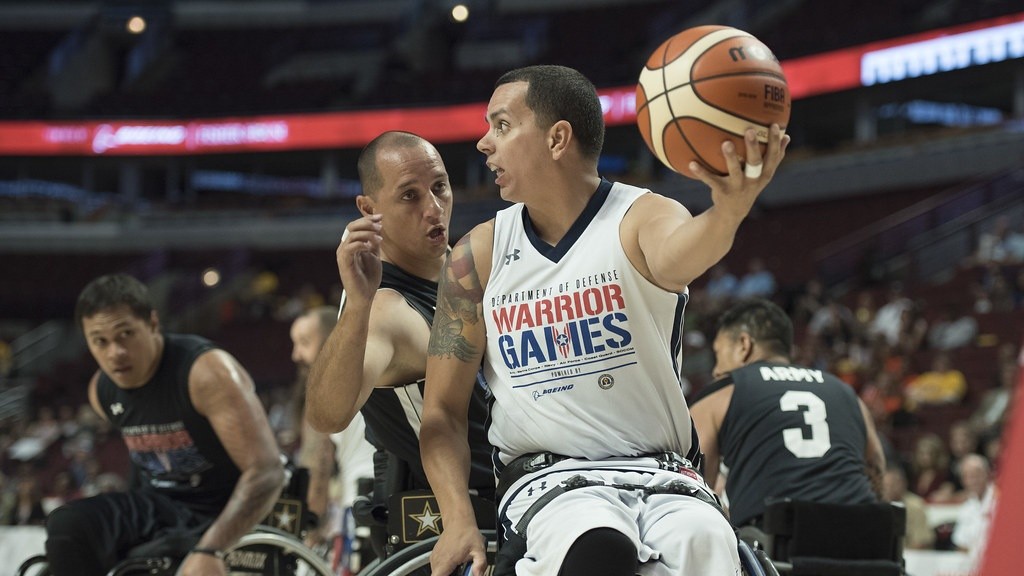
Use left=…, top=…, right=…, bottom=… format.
left=353, top=476, right=909, bottom=576
left=14, top=458, right=336, bottom=576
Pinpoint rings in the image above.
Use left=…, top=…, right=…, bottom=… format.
left=745, top=163, right=762, bottom=178
left=341, top=227, right=351, bottom=243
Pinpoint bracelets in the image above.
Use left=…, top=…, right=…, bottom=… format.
left=192, top=548, right=225, bottom=559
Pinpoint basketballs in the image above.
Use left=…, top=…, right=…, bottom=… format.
left=634, top=25, right=791, bottom=182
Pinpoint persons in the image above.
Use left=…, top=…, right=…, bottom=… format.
left=0, top=123, right=1024, bottom=576
left=419, top=65, right=791, bottom=576
